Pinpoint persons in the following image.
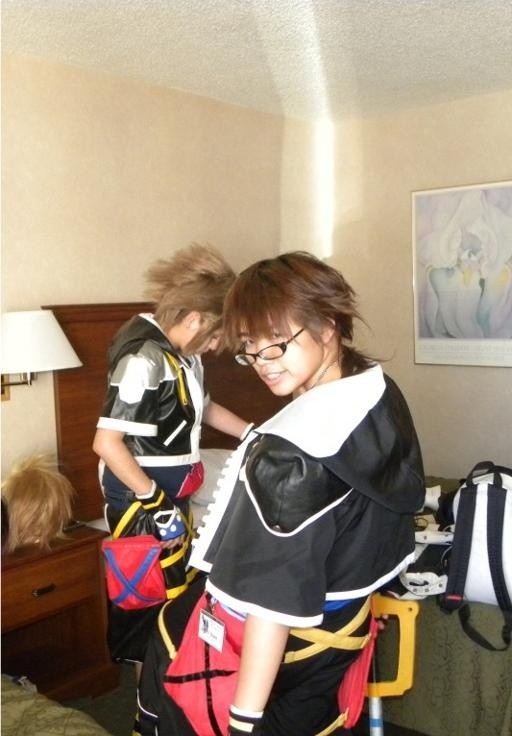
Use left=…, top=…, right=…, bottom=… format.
left=132, top=249, right=425, bottom=736
left=92, top=241, right=258, bottom=735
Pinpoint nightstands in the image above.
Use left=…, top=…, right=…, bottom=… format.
left=1, top=521, right=120, bottom=700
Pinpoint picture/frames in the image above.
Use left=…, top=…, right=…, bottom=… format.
left=412, top=182, right=512, bottom=368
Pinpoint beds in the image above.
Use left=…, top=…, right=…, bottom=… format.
left=42, top=291, right=511, bottom=736
left=1, top=674, right=111, bottom=736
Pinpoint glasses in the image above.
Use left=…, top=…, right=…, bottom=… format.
left=233, top=328, right=303, bottom=366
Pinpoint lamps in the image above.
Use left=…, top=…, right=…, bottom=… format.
left=1, top=308, right=82, bottom=400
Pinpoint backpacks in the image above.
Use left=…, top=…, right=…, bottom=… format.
left=436, top=455, right=512, bottom=654
